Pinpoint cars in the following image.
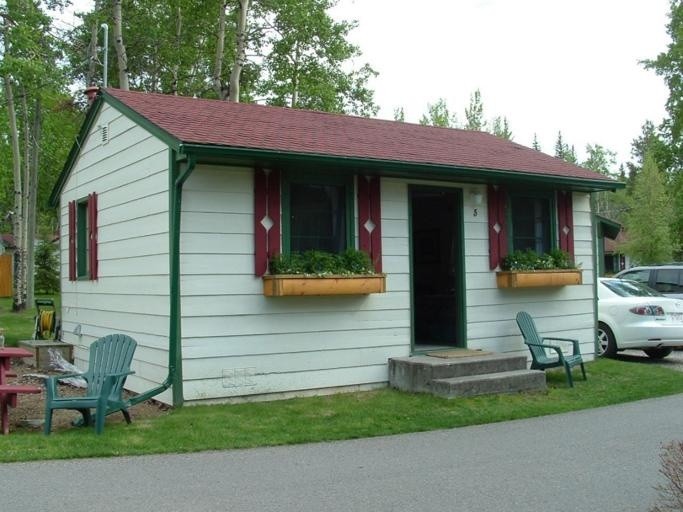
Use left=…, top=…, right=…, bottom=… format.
left=597, top=264, right=683, bottom=358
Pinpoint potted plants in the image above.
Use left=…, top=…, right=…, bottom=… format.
left=497, top=248, right=582, bottom=289
left=262, top=246, right=386, bottom=297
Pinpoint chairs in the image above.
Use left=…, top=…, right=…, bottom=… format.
left=43, top=334, right=137, bottom=434
left=514, top=310, right=587, bottom=387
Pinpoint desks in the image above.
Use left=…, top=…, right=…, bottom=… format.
left=0, top=345, right=42, bottom=434
